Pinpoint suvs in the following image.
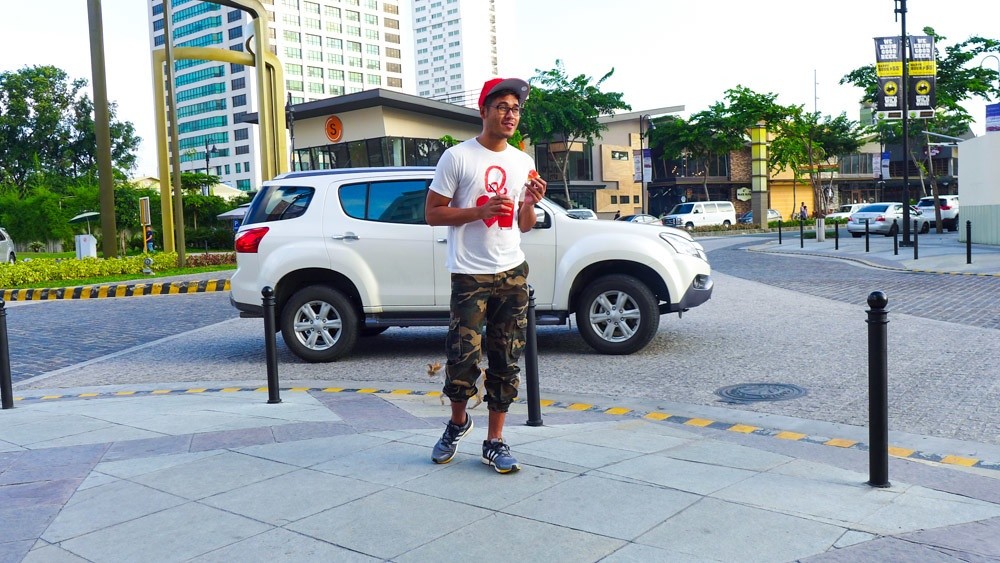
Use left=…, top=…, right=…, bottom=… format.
left=229, top=165, right=715, bottom=363
left=914, top=195, right=960, bottom=232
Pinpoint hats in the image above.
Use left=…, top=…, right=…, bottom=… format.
left=478, top=77, right=532, bottom=108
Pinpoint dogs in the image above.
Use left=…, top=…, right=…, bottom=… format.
left=428, top=362, right=520, bottom=410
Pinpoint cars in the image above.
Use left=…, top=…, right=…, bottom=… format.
left=614, top=214, right=664, bottom=226
left=737, top=209, right=783, bottom=225
left=824, top=203, right=868, bottom=221
left=566, top=209, right=598, bottom=219
left=0, top=227, right=17, bottom=265
left=846, top=202, right=931, bottom=238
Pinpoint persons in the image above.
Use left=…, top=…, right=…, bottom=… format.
left=800, top=202, right=808, bottom=220
left=614, top=211, right=620, bottom=220
left=425, top=78, right=547, bottom=473
left=146, top=226, right=153, bottom=251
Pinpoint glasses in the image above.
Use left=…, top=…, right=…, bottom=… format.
left=485, top=103, right=522, bottom=114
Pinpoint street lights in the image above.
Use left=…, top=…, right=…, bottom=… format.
left=640, top=114, right=657, bottom=216
left=204, top=137, right=219, bottom=196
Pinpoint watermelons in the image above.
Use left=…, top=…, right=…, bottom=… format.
left=528, top=170, right=541, bottom=180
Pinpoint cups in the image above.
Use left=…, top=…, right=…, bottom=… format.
left=498, top=195, right=515, bottom=230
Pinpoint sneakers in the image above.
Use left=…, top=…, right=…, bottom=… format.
left=481, top=439, right=522, bottom=473
left=431, top=412, right=474, bottom=463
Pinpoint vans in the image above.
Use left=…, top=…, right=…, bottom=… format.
left=660, top=201, right=737, bottom=231
